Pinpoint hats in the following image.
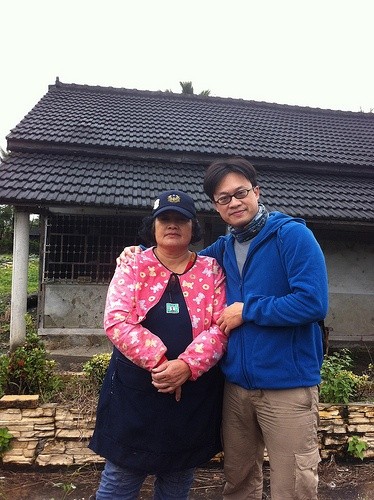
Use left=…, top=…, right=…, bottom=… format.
left=151, top=189, right=197, bottom=222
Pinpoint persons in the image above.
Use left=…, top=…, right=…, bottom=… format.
left=89, top=190, right=233, bottom=500
left=118, top=158, right=331, bottom=500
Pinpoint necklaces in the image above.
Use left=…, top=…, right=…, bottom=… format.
left=220, top=202, right=271, bottom=244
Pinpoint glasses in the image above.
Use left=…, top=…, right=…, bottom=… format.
left=213, top=187, right=254, bottom=205
